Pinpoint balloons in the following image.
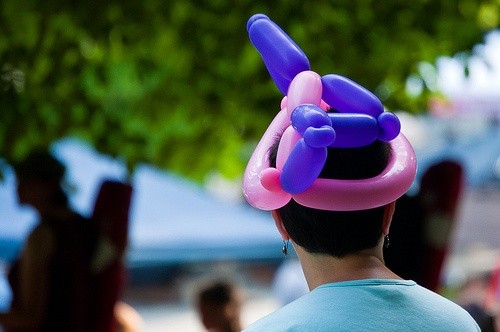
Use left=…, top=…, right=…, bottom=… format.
left=243, top=9, right=417, bottom=213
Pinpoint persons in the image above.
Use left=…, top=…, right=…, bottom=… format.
left=236, top=104, right=481, bottom=332
left=0, top=149, right=146, bottom=332
left=195, top=278, right=245, bottom=332
